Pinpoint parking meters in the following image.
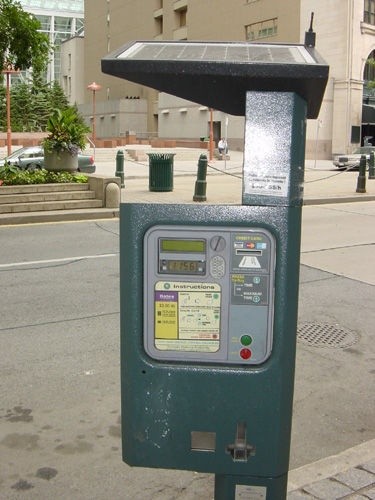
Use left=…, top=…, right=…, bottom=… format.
left=119, top=200, right=301, bottom=486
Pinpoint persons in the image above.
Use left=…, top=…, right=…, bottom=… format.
left=218, top=137, right=228, bottom=154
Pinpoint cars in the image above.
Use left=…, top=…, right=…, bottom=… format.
left=333, top=146, right=375, bottom=170
left=0, top=145, right=95, bottom=173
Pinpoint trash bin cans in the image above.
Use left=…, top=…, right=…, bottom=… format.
left=147, top=153, right=176, bottom=192
